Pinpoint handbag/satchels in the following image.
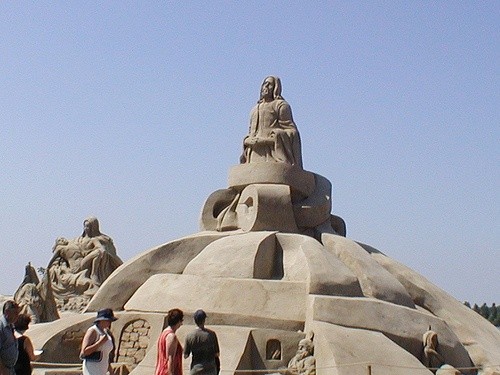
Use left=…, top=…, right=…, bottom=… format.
left=79, top=350, right=104, bottom=362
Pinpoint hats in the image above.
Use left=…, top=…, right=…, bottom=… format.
left=94, top=308, right=119, bottom=323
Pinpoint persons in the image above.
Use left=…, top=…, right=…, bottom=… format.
left=421, top=330, right=465, bottom=375
left=239, top=73, right=304, bottom=169
left=183, top=309, right=222, bottom=375
left=155, top=309, right=186, bottom=375
left=7, top=216, right=121, bottom=324
left=79, top=307, right=120, bottom=375
left=0, top=300, right=20, bottom=375
left=12, top=314, right=42, bottom=375
left=282, top=332, right=316, bottom=375
